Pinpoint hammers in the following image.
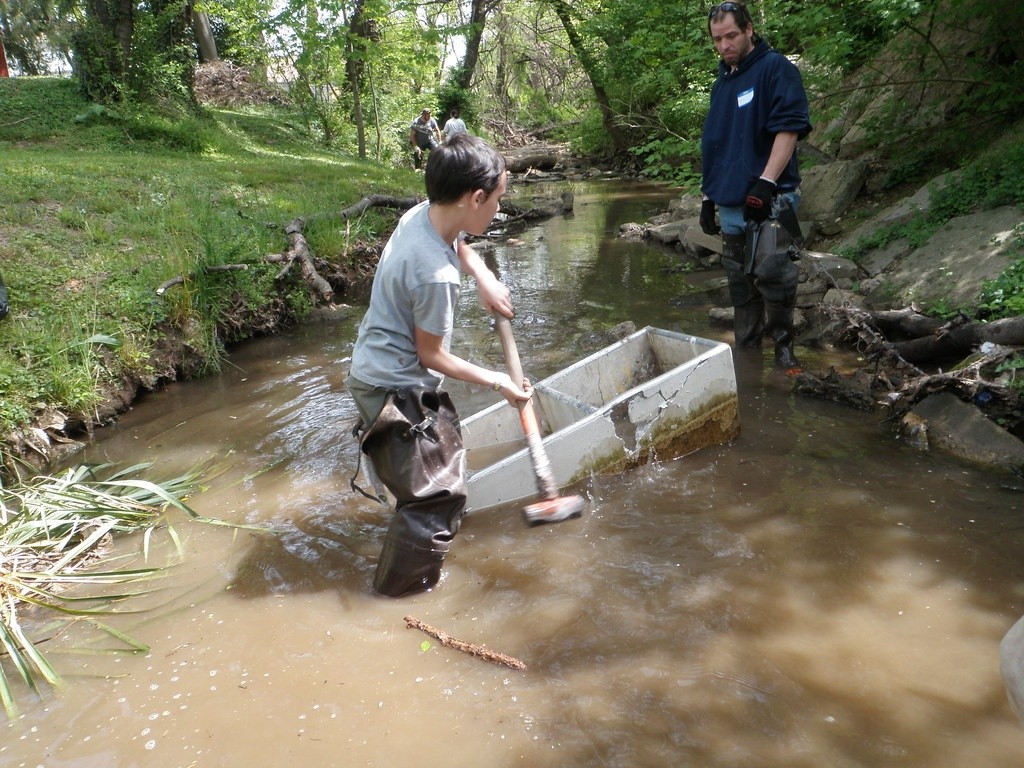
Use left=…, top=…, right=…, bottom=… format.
left=492, top=307, right=586, bottom=526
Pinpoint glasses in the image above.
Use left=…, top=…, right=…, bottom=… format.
left=709, top=1, right=748, bottom=18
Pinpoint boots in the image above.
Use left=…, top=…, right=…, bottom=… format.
left=718, top=252, right=801, bottom=371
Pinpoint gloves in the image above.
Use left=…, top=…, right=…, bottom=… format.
left=698, top=198, right=721, bottom=236
left=743, top=178, right=777, bottom=224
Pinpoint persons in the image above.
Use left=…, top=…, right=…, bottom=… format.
left=408, top=107, right=442, bottom=172
left=696, top=1, right=813, bottom=377
left=342, top=133, right=535, bottom=600
left=440, top=109, right=468, bottom=143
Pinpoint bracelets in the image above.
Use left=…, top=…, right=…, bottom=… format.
left=491, top=370, right=504, bottom=392
